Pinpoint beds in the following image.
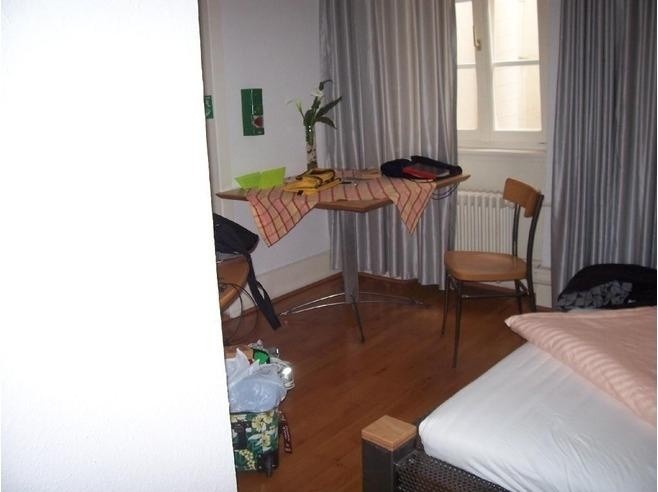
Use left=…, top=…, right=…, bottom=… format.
left=361, top=308, right=657, bottom=491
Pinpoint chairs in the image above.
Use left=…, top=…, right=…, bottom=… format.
left=442, top=177, right=544, bottom=367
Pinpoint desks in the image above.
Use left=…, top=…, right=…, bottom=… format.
left=216, top=174, right=471, bottom=342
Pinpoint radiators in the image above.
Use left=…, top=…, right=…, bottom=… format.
left=456, top=189, right=516, bottom=256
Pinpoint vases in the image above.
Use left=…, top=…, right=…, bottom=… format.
left=306, top=125, right=317, bottom=171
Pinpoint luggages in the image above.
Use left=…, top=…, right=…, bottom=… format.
left=223, top=346, right=278, bottom=477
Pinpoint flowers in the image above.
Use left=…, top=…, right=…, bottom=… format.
left=286, top=78, right=345, bottom=145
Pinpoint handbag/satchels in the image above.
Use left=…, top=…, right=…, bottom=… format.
left=212, top=213, right=258, bottom=259
left=381, top=155, right=462, bottom=180
left=285, top=168, right=342, bottom=195
